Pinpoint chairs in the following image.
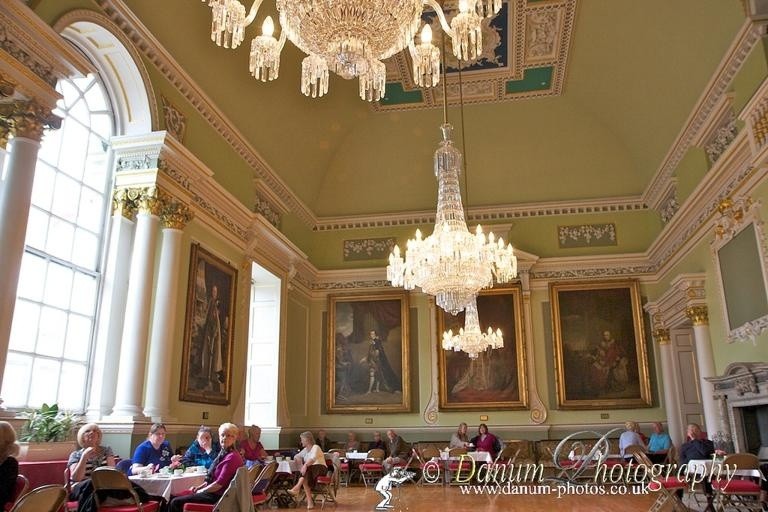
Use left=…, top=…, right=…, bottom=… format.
left=1, top=436, right=768, bottom=512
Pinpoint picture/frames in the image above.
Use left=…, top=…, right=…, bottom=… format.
left=177, top=240, right=239, bottom=406
left=429, top=280, right=531, bottom=412
left=324, top=289, right=413, bottom=414
left=546, top=276, right=654, bottom=413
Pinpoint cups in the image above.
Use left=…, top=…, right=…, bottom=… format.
left=276, top=456, right=282, bottom=462
left=267, top=455, right=273, bottom=461
left=174, top=469, right=180, bottom=475
left=286, top=457, right=290, bottom=462
left=354, top=449, right=357, bottom=453
left=469, top=442, right=473, bottom=445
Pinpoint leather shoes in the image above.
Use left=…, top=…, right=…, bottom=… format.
left=307, top=501, right=314, bottom=509
left=287, top=489, right=299, bottom=498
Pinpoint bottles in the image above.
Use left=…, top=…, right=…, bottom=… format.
left=186, top=465, right=204, bottom=473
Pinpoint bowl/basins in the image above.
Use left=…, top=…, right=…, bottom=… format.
left=156, top=473, right=171, bottom=477
left=440, top=451, right=449, bottom=460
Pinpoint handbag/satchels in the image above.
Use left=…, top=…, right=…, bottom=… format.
left=274, top=493, right=292, bottom=508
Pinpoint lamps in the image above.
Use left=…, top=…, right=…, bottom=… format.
left=199, top=0, right=508, bottom=106
left=383, top=42, right=519, bottom=317
left=440, top=58, right=504, bottom=363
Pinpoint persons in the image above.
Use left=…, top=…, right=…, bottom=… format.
left=197, top=285, right=229, bottom=393
left=671, top=422, right=718, bottom=512
left=647, top=422, right=672, bottom=465
left=588, top=330, right=627, bottom=396
left=618, top=421, right=646, bottom=463
left=0, top=421, right=21, bottom=512
left=366, top=330, right=384, bottom=395
left=635, top=424, right=647, bottom=446
